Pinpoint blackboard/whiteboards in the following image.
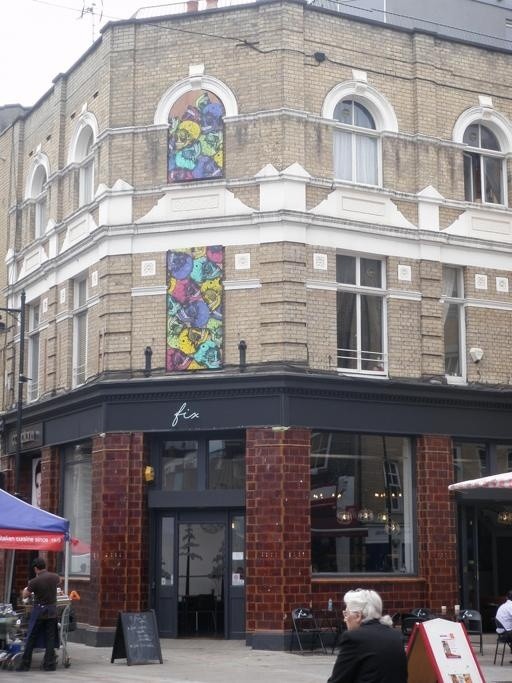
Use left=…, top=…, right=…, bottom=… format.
left=420, top=617, right=486, bottom=683
left=121, top=612, right=163, bottom=660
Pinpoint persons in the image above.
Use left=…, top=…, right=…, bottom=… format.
left=327, top=588, right=407, bottom=683
left=496, top=590, right=512, bottom=644
left=16, top=558, right=62, bottom=671
left=35, top=459, right=41, bottom=508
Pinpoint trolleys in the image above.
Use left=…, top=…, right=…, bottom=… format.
left=0, top=590, right=81, bottom=670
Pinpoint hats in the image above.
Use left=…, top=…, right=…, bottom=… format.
left=31, top=558, right=45, bottom=566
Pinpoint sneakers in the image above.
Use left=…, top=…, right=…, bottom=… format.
left=16, top=660, right=56, bottom=671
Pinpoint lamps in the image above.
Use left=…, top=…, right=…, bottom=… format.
left=312, top=489, right=402, bottom=536
left=145, top=466, right=156, bottom=482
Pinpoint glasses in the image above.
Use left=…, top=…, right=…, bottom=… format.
left=342, top=610, right=360, bottom=618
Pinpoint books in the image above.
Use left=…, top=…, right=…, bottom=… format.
left=57, top=588, right=72, bottom=601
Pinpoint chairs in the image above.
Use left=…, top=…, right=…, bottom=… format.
left=181, top=593, right=221, bottom=636
left=291, top=608, right=327, bottom=654
left=392, top=612, right=484, bottom=656
left=493, top=617, right=512, bottom=666
left=310, top=609, right=347, bottom=655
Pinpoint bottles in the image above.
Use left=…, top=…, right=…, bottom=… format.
left=327, top=597, right=334, bottom=611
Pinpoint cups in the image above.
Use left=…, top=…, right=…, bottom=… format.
left=441, top=605, right=460, bottom=615
